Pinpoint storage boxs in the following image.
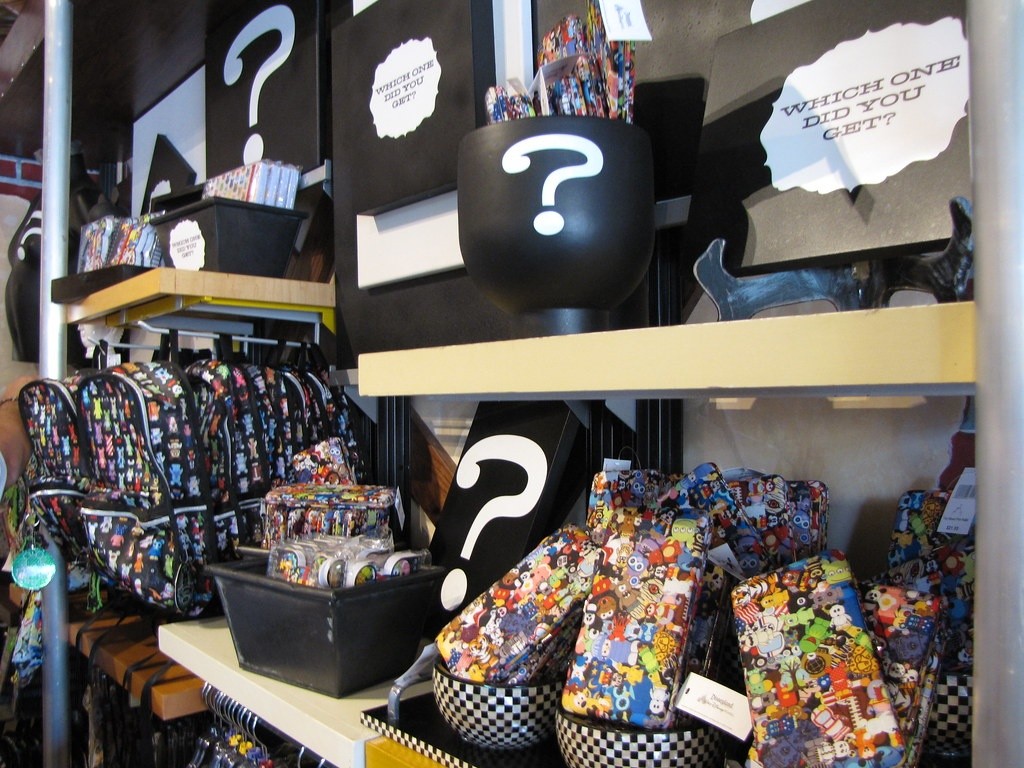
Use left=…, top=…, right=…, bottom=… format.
left=149, top=196, right=315, bottom=278
left=51, top=264, right=152, bottom=303
left=205, top=560, right=446, bottom=698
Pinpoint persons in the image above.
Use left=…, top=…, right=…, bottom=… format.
left=0, top=375, right=39, bottom=573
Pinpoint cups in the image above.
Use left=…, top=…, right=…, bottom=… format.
left=456, top=114, right=653, bottom=336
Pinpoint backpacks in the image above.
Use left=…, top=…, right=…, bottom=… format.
left=17, top=369, right=95, bottom=566
left=184, top=331, right=369, bottom=616
left=77, top=327, right=217, bottom=619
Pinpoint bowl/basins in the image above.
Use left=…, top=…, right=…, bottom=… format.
left=431, top=652, right=567, bottom=752
left=554, top=701, right=719, bottom=768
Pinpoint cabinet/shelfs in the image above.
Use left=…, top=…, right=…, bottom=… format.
left=7, top=266, right=978, bottom=768
